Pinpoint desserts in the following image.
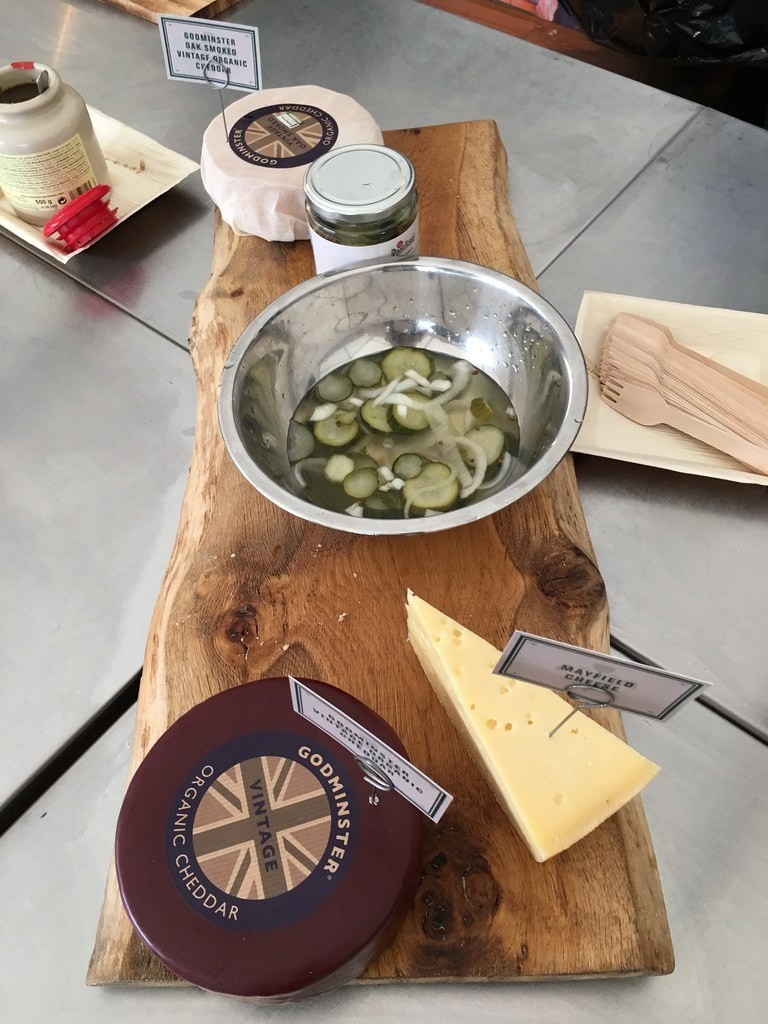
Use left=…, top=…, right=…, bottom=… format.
left=397, top=587, right=662, bottom=863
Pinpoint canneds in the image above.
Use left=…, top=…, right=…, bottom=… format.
left=302, top=143, right=426, bottom=273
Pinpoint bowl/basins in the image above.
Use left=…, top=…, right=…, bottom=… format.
left=215, top=256, right=590, bottom=535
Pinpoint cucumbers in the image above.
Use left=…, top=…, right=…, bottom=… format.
left=289, top=344, right=522, bottom=516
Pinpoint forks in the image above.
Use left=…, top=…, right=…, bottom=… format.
left=598, top=312, right=767, bottom=477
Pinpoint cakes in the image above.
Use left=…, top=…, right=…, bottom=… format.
left=112, top=679, right=421, bottom=1006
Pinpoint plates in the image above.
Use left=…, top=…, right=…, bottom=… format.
left=565, top=289, right=768, bottom=487
